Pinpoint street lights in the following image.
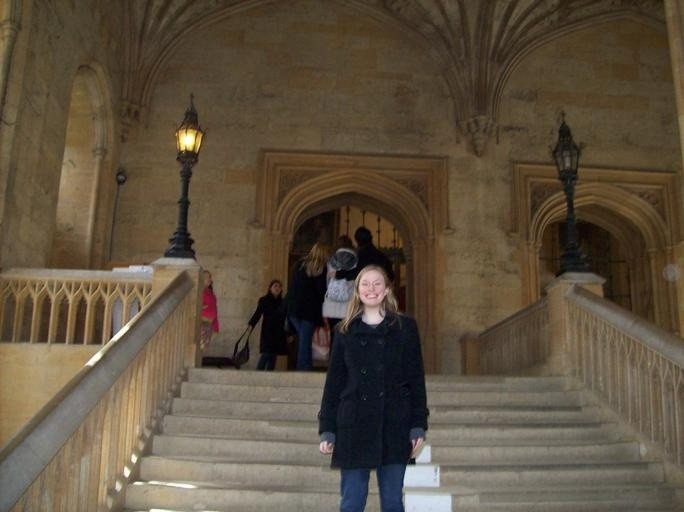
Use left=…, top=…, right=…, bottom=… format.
left=164, top=91, right=205, bottom=258
left=552, top=111, right=581, bottom=276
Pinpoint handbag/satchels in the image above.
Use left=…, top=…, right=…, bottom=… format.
left=232, top=336, right=250, bottom=368
left=309, top=326, right=335, bottom=363
left=326, top=276, right=355, bottom=302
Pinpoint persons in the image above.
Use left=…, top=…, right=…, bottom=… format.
left=200, top=270, right=219, bottom=358
left=287, top=226, right=397, bottom=371
left=248, top=280, right=283, bottom=371
left=318, top=264, right=427, bottom=512
